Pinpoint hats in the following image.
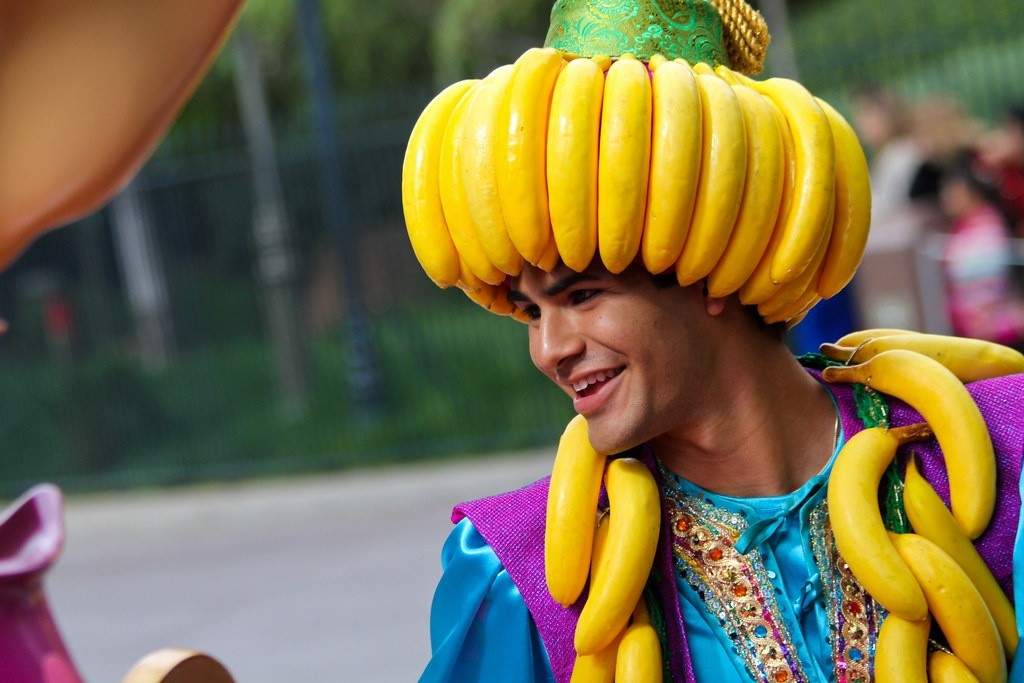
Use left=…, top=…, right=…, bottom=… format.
left=402, top=0, right=871, bottom=331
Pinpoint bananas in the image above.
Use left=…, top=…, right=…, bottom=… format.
left=820, top=328, right=1024, bottom=683
left=402, top=50, right=871, bottom=326
left=543, top=411, right=664, bottom=683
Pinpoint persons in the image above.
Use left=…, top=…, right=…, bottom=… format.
left=419, top=58, right=1024, bottom=683
